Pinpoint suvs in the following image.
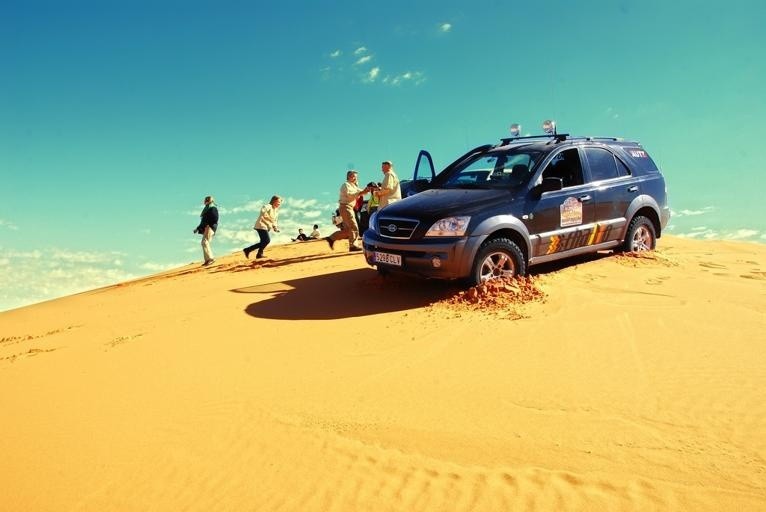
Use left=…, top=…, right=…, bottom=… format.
left=361, top=118, right=671, bottom=295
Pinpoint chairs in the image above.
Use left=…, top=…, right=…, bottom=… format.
left=510, top=164, right=529, bottom=178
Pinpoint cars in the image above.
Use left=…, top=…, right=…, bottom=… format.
left=330, top=174, right=434, bottom=239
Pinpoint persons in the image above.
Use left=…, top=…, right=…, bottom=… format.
left=194, top=196, right=218, bottom=266
left=325, top=161, right=402, bottom=252
left=244, top=195, right=282, bottom=259
left=290, top=225, right=320, bottom=241
